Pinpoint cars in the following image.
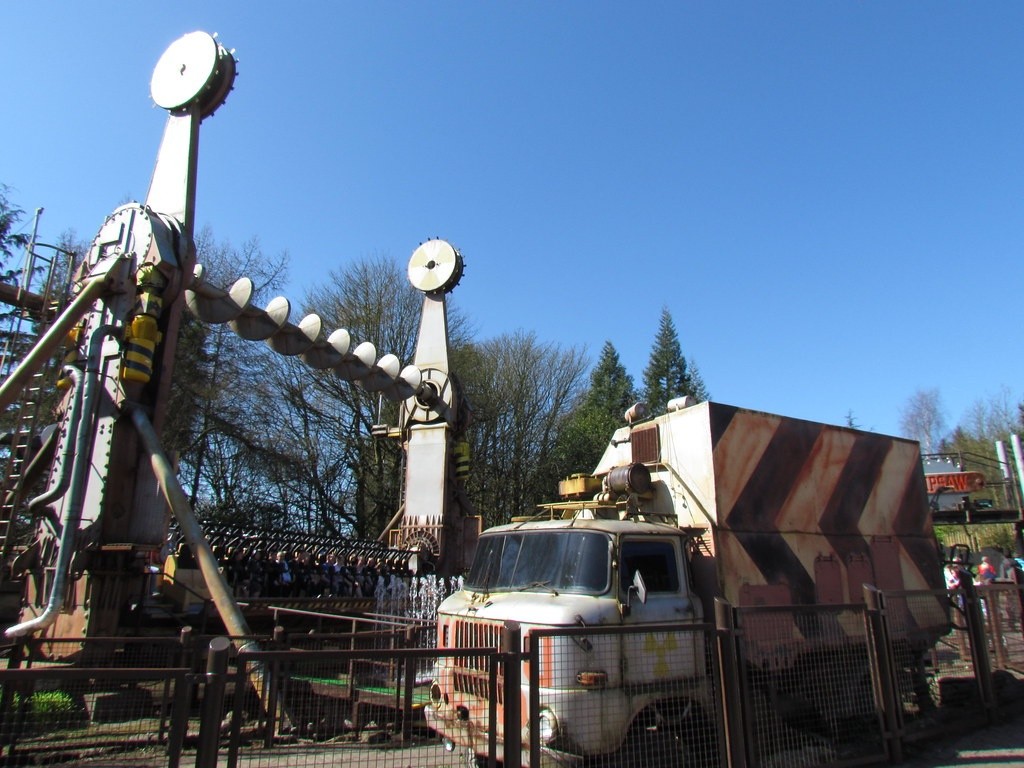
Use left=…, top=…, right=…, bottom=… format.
left=974, top=498, right=992, bottom=508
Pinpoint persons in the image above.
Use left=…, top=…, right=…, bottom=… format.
left=943, top=557, right=986, bottom=615
left=979, top=556, right=997, bottom=580
left=165, top=528, right=435, bottom=600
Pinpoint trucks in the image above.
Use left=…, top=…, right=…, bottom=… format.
left=423, top=395, right=953, bottom=766
